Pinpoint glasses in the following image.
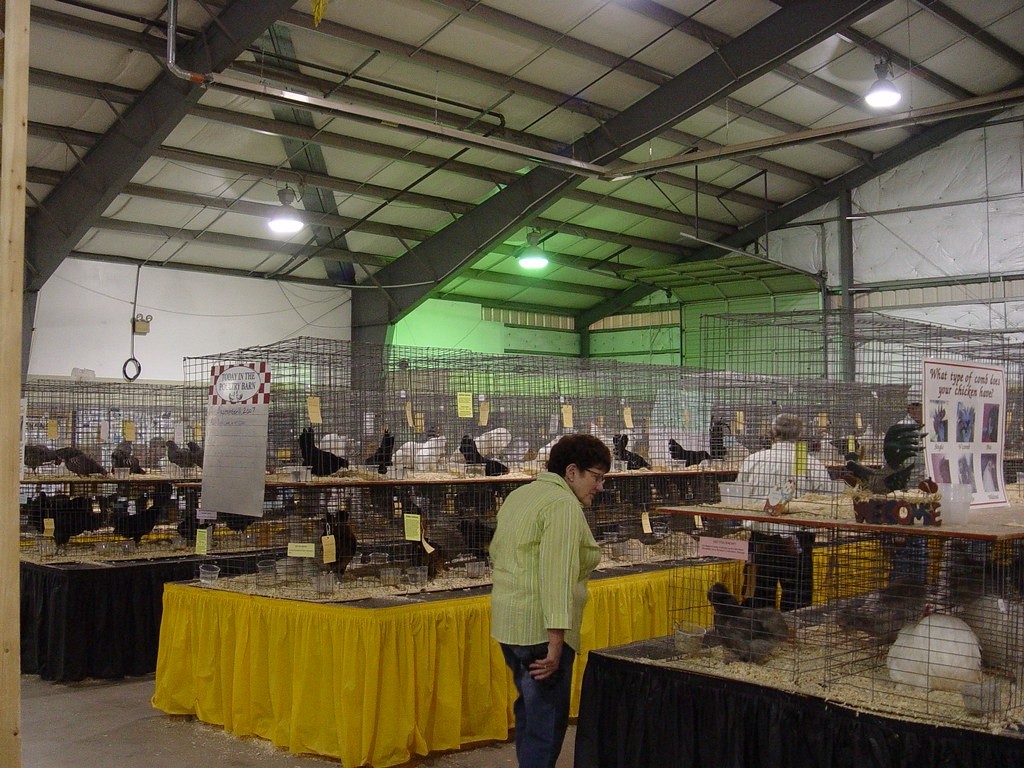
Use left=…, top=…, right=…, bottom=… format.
left=584, top=468, right=607, bottom=482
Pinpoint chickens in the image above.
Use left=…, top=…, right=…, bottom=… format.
left=736, top=404, right=888, bottom=459
left=19, top=437, right=280, bottom=553
left=698, top=576, right=1024, bottom=692
left=300, top=414, right=749, bottom=586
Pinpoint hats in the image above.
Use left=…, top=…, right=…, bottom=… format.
left=907, top=384, right=923, bottom=406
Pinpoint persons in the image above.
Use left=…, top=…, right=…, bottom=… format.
left=490, top=434, right=611, bottom=768
left=881, top=403, right=929, bottom=584
left=734, top=413, right=832, bottom=612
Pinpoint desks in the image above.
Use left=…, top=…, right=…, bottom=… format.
left=19, top=523, right=494, bottom=683
left=150, top=524, right=939, bottom=766
left=572, top=598, right=1024, bottom=767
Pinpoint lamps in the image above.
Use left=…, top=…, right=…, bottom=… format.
left=864, top=63, right=902, bottom=108
left=267, top=187, right=304, bottom=233
left=518, top=232, right=548, bottom=268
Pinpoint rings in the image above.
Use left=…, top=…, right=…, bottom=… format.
left=546, top=665, right=551, bottom=672
